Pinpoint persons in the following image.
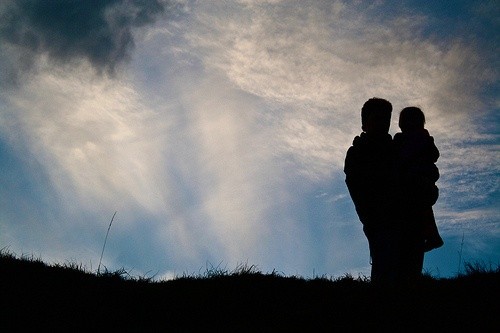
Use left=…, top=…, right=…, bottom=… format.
left=343, top=98, right=444, bottom=284
left=392, top=106, right=440, bottom=207
